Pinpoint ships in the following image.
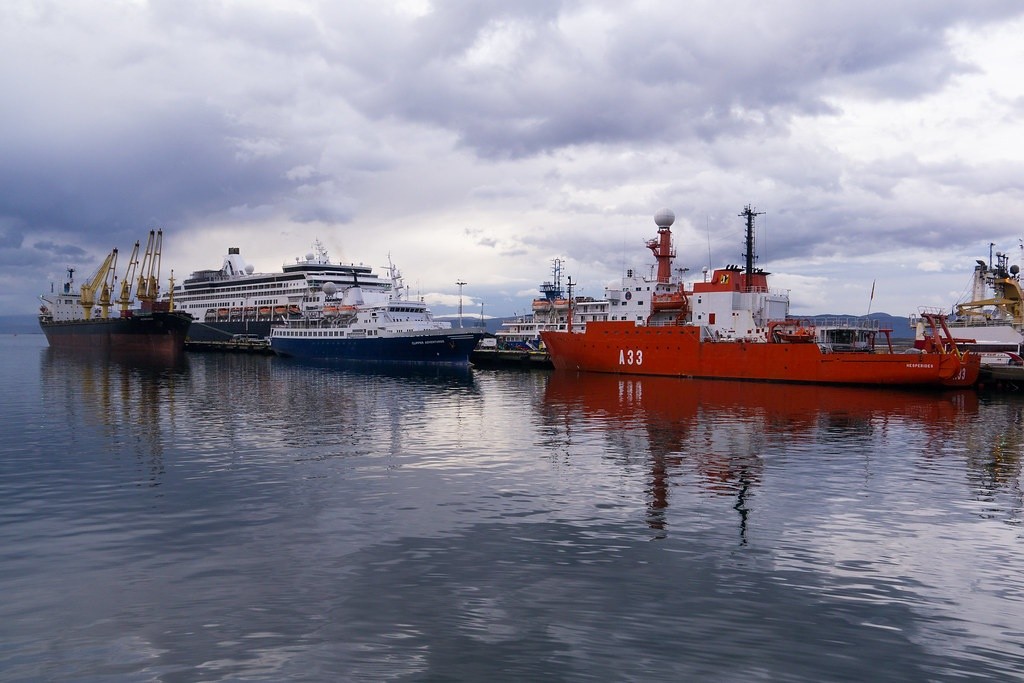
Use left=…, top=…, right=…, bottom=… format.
left=157, top=247, right=453, bottom=355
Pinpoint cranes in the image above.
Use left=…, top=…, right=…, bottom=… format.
left=79, top=227, right=163, bottom=321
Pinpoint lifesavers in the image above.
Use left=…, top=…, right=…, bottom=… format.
left=753, top=337, right=758, bottom=342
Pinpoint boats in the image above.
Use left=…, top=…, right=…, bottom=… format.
left=231, top=307, right=241, bottom=318
left=244, top=306, right=256, bottom=317
left=269, top=325, right=488, bottom=365
left=37, top=263, right=200, bottom=350
left=474, top=259, right=656, bottom=364
left=538, top=201, right=981, bottom=389
left=218, top=308, right=228, bottom=318
left=776, top=329, right=818, bottom=343
left=322, top=307, right=340, bottom=317
left=339, top=306, right=357, bottom=316
left=204, top=309, right=216, bottom=318
left=259, top=306, right=270, bottom=315
left=909, top=242, right=1024, bottom=384
left=288, top=306, right=301, bottom=315
left=275, top=306, right=287, bottom=314
left=651, top=294, right=685, bottom=309
